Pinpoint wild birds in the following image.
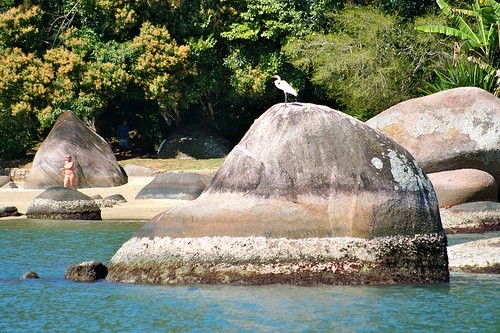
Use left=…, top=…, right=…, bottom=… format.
left=270, top=74, right=299, bottom=108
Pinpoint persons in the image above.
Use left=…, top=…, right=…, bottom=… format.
left=61, top=154, right=76, bottom=190
left=116, top=121, right=129, bottom=159
